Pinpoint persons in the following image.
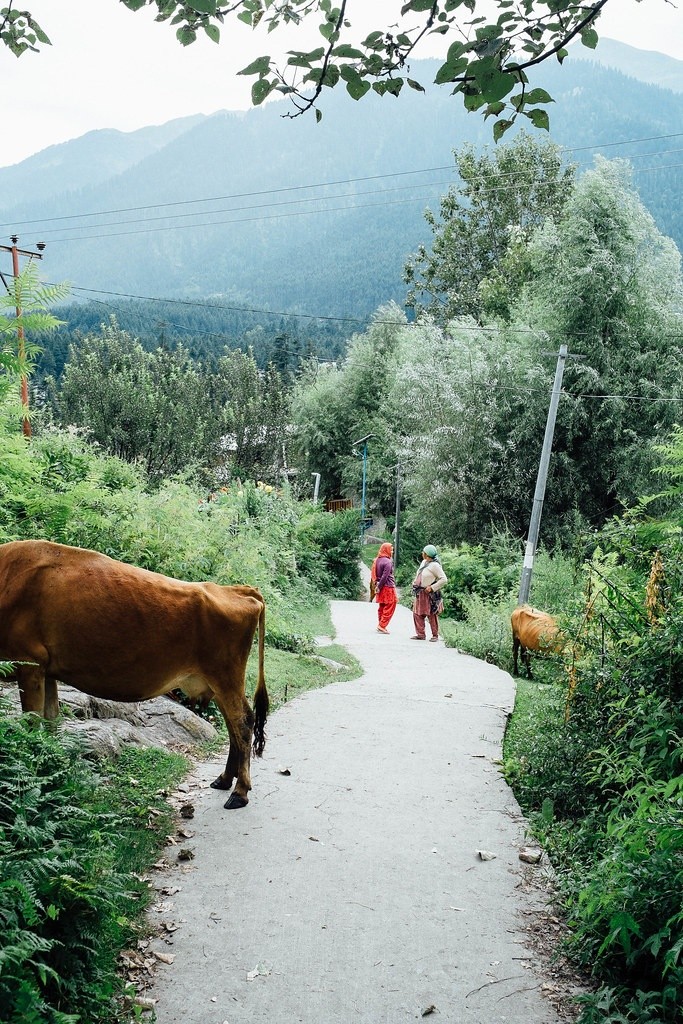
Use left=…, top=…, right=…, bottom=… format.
left=371, top=542, right=397, bottom=635
left=410, top=544, right=448, bottom=642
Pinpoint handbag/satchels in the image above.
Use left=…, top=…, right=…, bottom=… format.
left=432, top=598, right=444, bottom=616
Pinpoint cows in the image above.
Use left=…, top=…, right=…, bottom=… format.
left=510, top=604, right=569, bottom=677
left=1, top=540, right=270, bottom=809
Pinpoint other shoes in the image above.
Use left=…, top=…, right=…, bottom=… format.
left=377, top=626, right=390, bottom=634
left=410, top=635, right=425, bottom=640
left=429, top=637, right=438, bottom=642
